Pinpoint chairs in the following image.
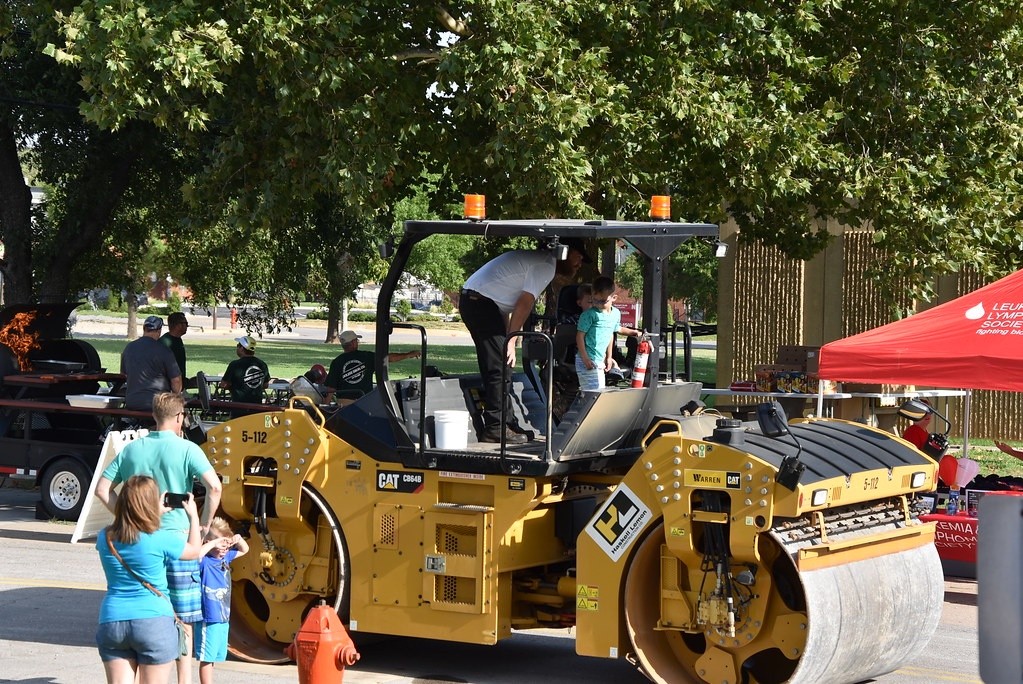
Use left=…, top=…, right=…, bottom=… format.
left=188, top=372, right=292, bottom=421
left=549, top=283, right=581, bottom=373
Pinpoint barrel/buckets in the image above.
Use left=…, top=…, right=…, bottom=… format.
left=434, top=411, right=470, bottom=449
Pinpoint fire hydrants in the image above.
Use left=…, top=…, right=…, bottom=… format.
left=229, top=308, right=238, bottom=330
left=282, top=598, right=362, bottom=684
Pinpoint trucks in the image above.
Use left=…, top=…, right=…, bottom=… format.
left=0, top=302, right=229, bottom=523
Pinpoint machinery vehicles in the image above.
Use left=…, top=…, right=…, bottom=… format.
left=191, top=193, right=944, bottom=684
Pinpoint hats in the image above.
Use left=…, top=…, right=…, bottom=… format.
left=340, top=330, right=363, bottom=343
left=143, top=316, right=164, bottom=330
left=568, top=238, right=591, bottom=263
left=234, top=336, right=256, bottom=352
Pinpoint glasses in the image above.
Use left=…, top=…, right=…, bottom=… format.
left=173, top=411, right=187, bottom=419
left=593, top=298, right=611, bottom=305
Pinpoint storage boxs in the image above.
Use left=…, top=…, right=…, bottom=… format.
left=966, top=490, right=991, bottom=518
left=754, top=364, right=801, bottom=377
left=778, top=345, right=819, bottom=375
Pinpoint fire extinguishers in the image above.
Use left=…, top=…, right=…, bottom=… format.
left=632, top=329, right=660, bottom=388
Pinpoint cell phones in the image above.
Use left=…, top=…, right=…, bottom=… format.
left=163, top=494, right=190, bottom=508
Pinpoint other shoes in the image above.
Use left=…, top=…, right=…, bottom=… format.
left=482, top=422, right=536, bottom=444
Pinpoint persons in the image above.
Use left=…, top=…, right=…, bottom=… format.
left=95, top=475, right=202, bottom=684
left=901, top=397, right=931, bottom=452
left=220, top=337, right=273, bottom=420
left=459, top=237, right=584, bottom=444
left=120, top=312, right=189, bottom=414
left=554, top=276, right=643, bottom=392
left=198, top=517, right=249, bottom=684
left=95, top=391, right=222, bottom=684
left=994, top=440, right=1023, bottom=462
left=322, top=330, right=422, bottom=406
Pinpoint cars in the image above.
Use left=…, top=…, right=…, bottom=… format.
left=392, top=300, right=445, bottom=329
left=76, top=285, right=148, bottom=310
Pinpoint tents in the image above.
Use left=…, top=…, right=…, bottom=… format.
left=817, top=271, right=1023, bottom=458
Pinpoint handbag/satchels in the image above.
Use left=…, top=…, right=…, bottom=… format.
left=174, top=619, right=189, bottom=661
